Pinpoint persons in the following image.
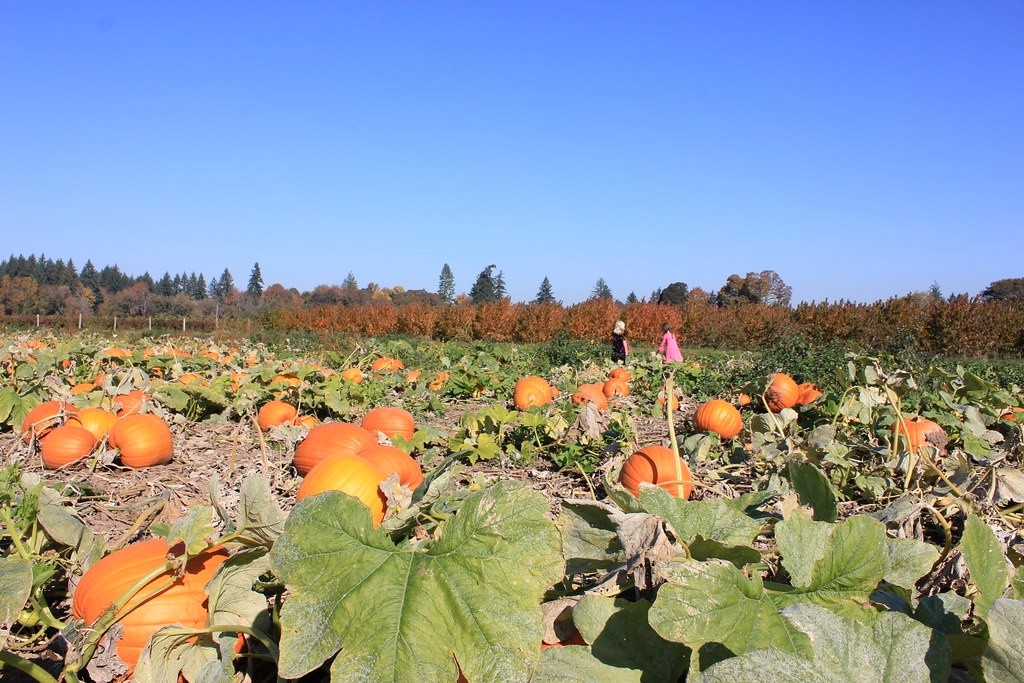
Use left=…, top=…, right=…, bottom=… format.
left=656, top=323, right=684, bottom=364
left=611, top=320, right=627, bottom=365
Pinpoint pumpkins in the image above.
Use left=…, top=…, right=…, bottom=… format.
left=2, top=334, right=1024, bottom=681
